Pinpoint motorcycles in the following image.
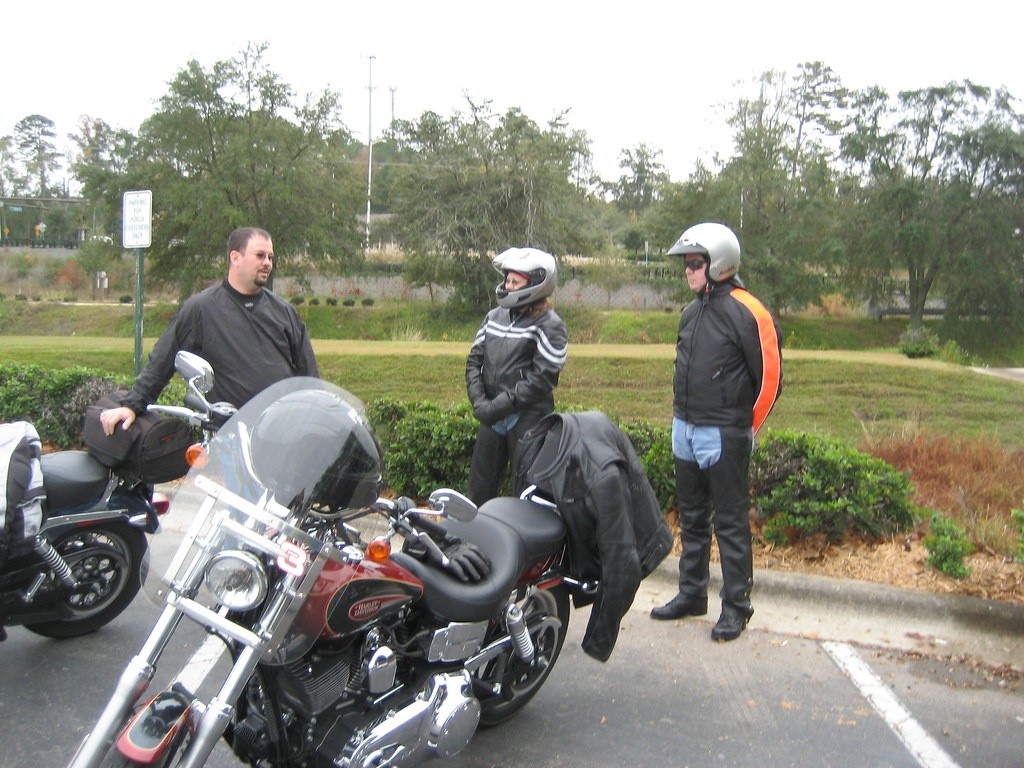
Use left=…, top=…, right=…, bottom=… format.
left=65, top=353, right=604, bottom=768
left=1, top=401, right=199, bottom=639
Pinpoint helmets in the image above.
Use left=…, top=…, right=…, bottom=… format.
left=496, top=248, right=557, bottom=308
left=665, top=223, right=742, bottom=284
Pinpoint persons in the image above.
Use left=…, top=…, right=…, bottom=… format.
left=465, top=246, right=568, bottom=509
left=650, top=222, right=782, bottom=641
left=101, top=228, right=321, bottom=552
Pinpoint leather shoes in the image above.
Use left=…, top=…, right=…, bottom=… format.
left=652, top=593, right=709, bottom=620
left=712, top=611, right=746, bottom=641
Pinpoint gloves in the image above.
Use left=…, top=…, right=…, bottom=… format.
left=403, top=526, right=490, bottom=583
left=474, top=393, right=513, bottom=426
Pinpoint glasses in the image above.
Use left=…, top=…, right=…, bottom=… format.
left=684, top=260, right=707, bottom=270
